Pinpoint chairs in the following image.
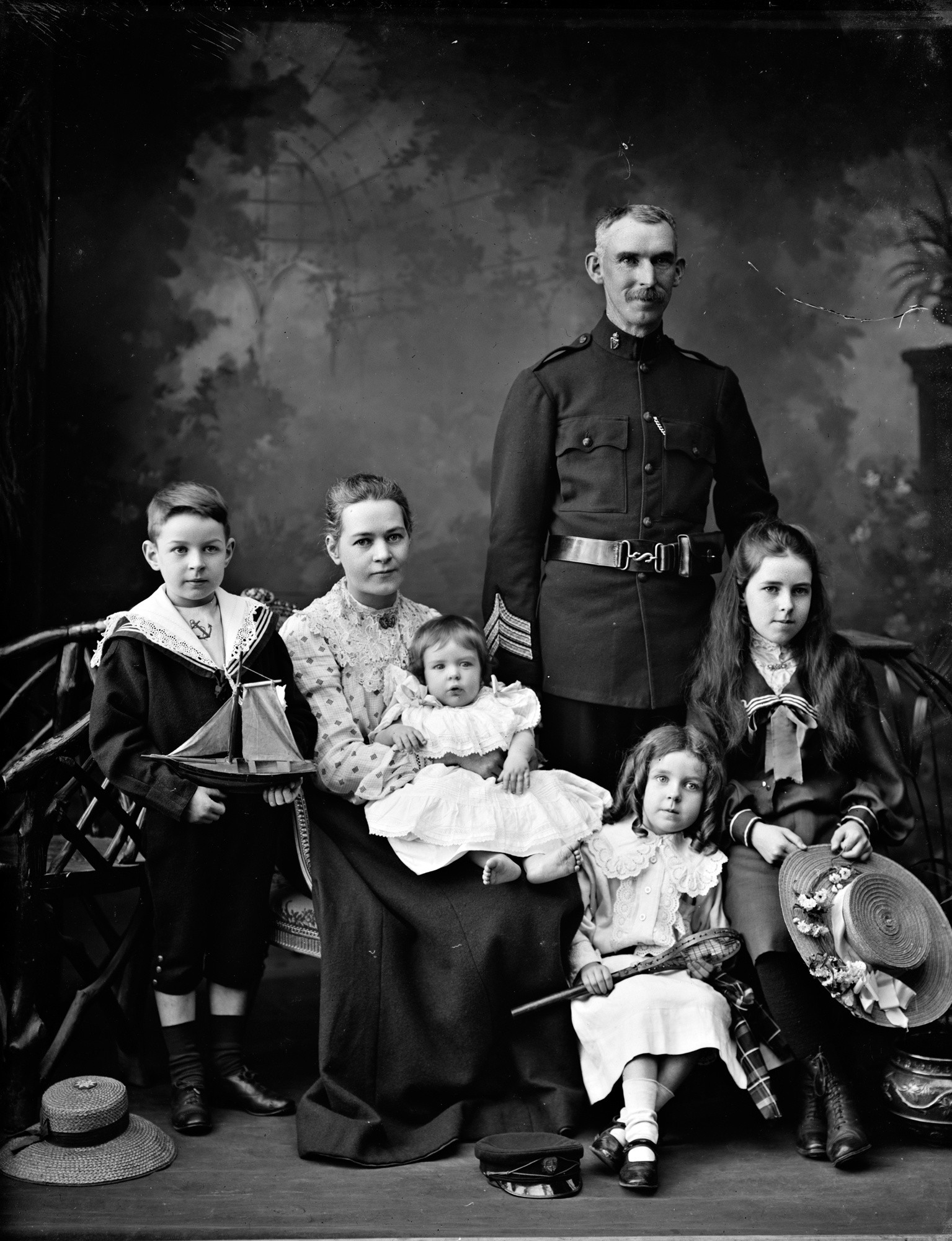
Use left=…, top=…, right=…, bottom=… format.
left=240, top=586, right=323, bottom=962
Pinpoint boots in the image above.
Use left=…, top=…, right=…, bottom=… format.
left=802, top=1043, right=872, bottom=1166
left=796, top=1070, right=828, bottom=1155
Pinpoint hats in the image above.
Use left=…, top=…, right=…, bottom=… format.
left=0, top=1075, right=177, bottom=1186
left=778, top=844, right=952, bottom=1033
left=474, top=1132, right=584, bottom=1198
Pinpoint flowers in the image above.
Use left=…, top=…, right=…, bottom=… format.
left=792, top=866, right=867, bottom=998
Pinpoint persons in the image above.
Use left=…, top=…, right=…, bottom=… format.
left=685, top=519, right=918, bottom=1167
left=363, top=614, right=612, bottom=885
left=482, top=204, right=781, bottom=829
left=89, top=479, right=318, bottom=1137
left=573, top=724, right=747, bottom=1188
left=278, top=474, right=585, bottom=1168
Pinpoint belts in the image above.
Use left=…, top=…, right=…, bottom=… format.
left=545, top=533, right=680, bottom=574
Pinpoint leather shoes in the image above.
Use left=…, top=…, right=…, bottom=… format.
left=169, top=1078, right=213, bottom=1131
left=209, top=1065, right=294, bottom=1116
left=619, top=1139, right=659, bottom=1187
left=590, top=1115, right=628, bottom=1171
